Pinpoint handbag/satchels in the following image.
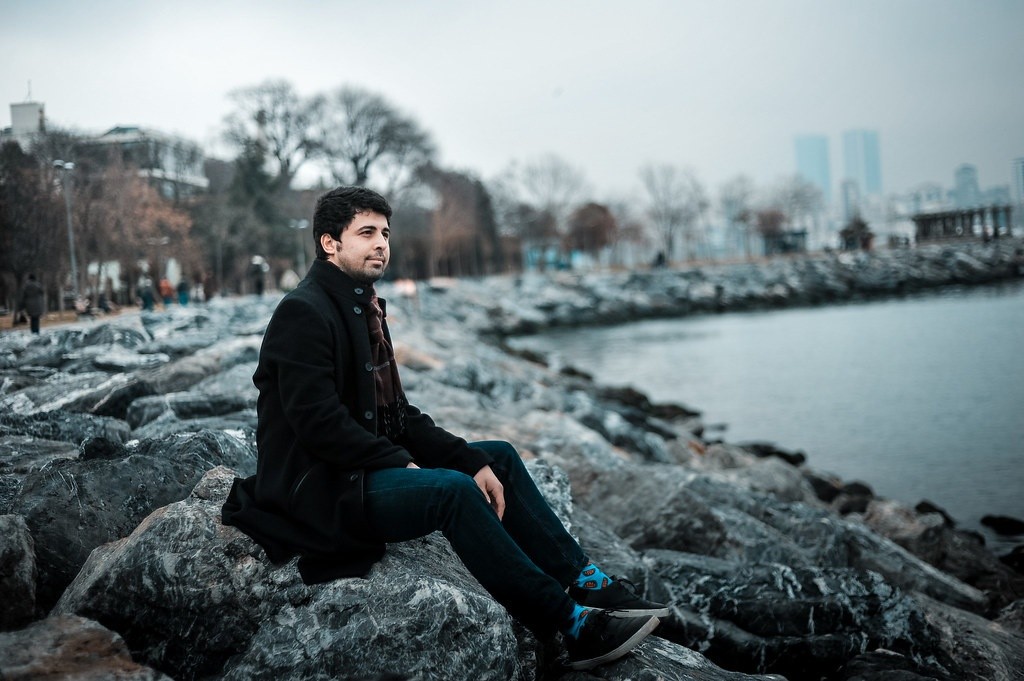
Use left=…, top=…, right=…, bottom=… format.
left=12, top=308, right=27, bottom=327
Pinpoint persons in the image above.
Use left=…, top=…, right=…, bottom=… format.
left=86, top=292, right=118, bottom=314
left=159, top=277, right=174, bottom=303
left=222, top=186, right=669, bottom=668
left=178, top=278, right=190, bottom=304
left=142, top=279, right=158, bottom=310
left=248, top=255, right=267, bottom=296
left=17, top=273, right=43, bottom=335
left=203, top=272, right=216, bottom=301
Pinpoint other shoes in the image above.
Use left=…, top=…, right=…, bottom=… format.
left=562, top=608, right=660, bottom=670
left=568, top=574, right=670, bottom=617
